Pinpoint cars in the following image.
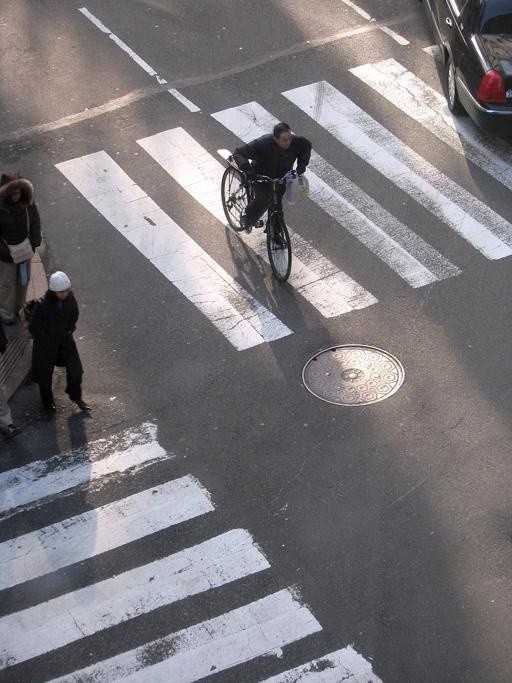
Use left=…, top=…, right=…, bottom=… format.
left=422, top=0, right=512, bottom=136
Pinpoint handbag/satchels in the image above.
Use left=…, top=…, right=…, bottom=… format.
left=9, top=239, right=35, bottom=264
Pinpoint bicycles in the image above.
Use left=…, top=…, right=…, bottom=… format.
left=221, top=154, right=304, bottom=283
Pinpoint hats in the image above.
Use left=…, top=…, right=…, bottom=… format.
left=50, top=270, right=70, bottom=291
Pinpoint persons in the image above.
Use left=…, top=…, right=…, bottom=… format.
left=23, top=271, right=94, bottom=413
left=2, top=173, right=42, bottom=326
left=234, top=121, right=313, bottom=234
left=0, top=312, right=22, bottom=438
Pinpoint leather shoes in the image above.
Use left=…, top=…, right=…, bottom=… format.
left=240, top=210, right=250, bottom=229
left=68, top=393, right=92, bottom=411
